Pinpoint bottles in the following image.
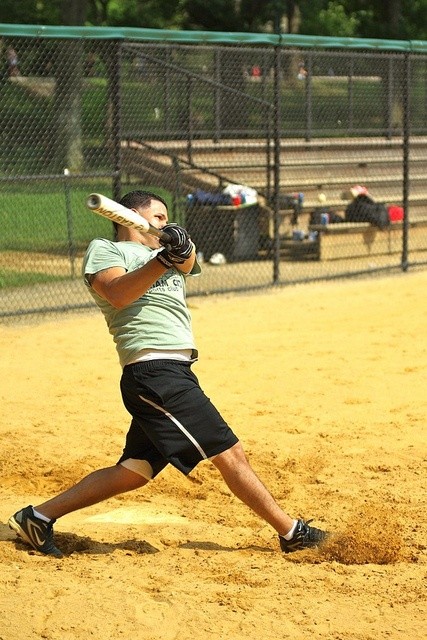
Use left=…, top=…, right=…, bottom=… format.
left=298, top=192, right=304, bottom=208
left=320, top=211, right=330, bottom=226
left=294, top=191, right=299, bottom=207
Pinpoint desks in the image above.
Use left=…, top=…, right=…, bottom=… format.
left=184, top=204, right=262, bottom=263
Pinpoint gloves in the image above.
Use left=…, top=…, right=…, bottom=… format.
left=160, top=227, right=188, bottom=251
left=158, top=235, right=193, bottom=269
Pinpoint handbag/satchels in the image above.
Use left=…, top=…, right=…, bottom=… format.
left=345, top=194, right=391, bottom=231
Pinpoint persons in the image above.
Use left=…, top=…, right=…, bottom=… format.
left=8, top=190, right=337, bottom=558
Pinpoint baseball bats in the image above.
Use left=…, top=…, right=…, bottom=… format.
left=84, top=192, right=172, bottom=244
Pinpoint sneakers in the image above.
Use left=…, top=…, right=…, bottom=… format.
left=278, top=519, right=330, bottom=553
left=8, top=505, right=63, bottom=558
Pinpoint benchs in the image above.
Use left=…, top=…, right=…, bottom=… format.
left=264, top=191, right=427, bottom=238
left=309, top=215, right=427, bottom=261
left=193, top=153, right=427, bottom=171
left=134, top=133, right=426, bottom=152
left=241, top=174, right=427, bottom=189
